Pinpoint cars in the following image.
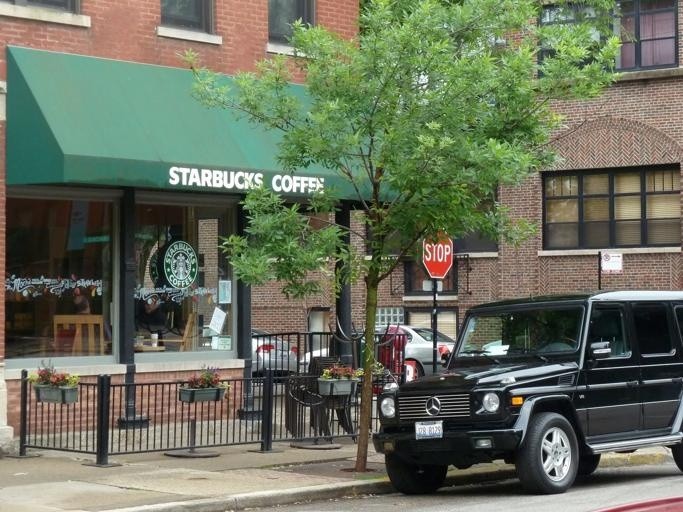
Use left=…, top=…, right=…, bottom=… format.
left=251, top=325, right=456, bottom=380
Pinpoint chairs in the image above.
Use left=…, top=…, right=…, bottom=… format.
left=41, top=313, right=194, bottom=356
left=284, top=357, right=358, bottom=444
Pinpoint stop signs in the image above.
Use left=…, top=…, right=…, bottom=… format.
left=421, top=228, right=454, bottom=279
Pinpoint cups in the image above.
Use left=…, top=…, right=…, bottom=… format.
left=150, top=331, right=158, bottom=347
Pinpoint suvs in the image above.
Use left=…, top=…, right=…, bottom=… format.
left=373, top=290, right=683, bottom=494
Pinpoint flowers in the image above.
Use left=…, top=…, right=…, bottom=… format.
left=189, top=363, right=232, bottom=388
left=25, top=360, right=80, bottom=387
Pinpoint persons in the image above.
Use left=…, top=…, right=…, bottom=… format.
left=162, top=303, right=176, bottom=329
left=70, top=286, right=90, bottom=314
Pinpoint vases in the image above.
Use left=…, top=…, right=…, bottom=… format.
left=178, top=388, right=227, bottom=403
left=32, top=385, right=78, bottom=404
